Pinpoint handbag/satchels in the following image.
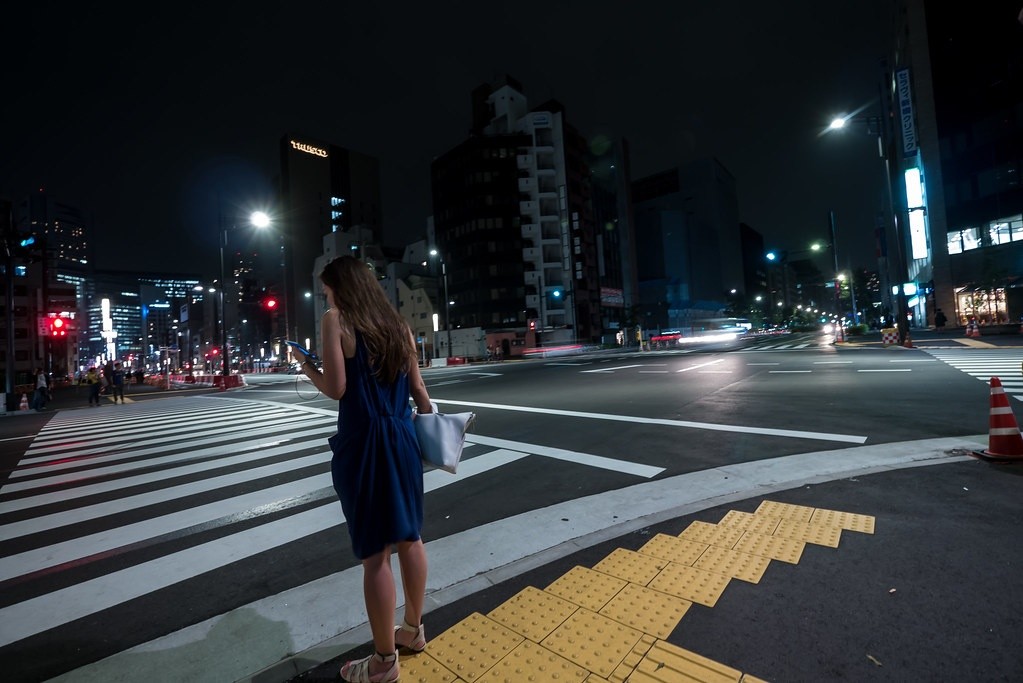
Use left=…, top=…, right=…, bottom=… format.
left=412, top=402, right=476, bottom=474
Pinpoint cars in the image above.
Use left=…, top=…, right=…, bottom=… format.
left=19, top=384, right=34, bottom=397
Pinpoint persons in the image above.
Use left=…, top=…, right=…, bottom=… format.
left=934, top=309, right=947, bottom=335
left=88, top=367, right=103, bottom=407
left=34, top=367, right=52, bottom=411
left=122, top=366, right=146, bottom=392
left=485, top=343, right=504, bottom=363
left=112, top=363, right=126, bottom=404
left=294, top=252, right=436, bottom=683
left=883, top=302, right=909, bottom=345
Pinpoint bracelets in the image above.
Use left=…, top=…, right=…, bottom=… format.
left=301, top=359, right=316, bottom=372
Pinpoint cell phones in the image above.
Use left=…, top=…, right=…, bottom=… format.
left=283, top=340, right=320, bottom=363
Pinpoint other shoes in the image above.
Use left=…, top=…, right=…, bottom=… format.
left=89, top=404, right=94, bottom=407
left=121, top=401, right=125, bottom=404
left=96, top=403, right=101, bottom=406
left=114, top=401, right=116, bottom=404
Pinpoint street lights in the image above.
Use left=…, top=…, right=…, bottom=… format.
left=430, top=248, right=452, bottom=358
left=840, top=269, right=857, bottom=322
left=252, top=211, right=289, bottom=338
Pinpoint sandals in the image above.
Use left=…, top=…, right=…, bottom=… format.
left=394, top=617, right=427, bottom=651
left=340, top=649, right=399, bottom=683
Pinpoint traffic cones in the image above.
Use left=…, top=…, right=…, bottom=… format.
left=638, top=340, right=644, bottom=352
left=904, top=332, right=913, bottom=348
left=971, top=375, right=1023, bottom=462
left=665, top=340, right=670, bottom=349
left=19, top=393, right=29, bottom=410
left=965, top=324, right=973, bottom=336
left=646, top=341, right=651, bottom=351
left=656, top=342, right=660, bottom=350
left=970, top=323, right=982, bottom=337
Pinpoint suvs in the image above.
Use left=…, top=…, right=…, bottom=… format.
left=54, top=375, right=71, bottom=390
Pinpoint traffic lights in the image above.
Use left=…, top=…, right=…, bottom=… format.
left=554, top=290, right=559, bottom=301
left=767, top=246, right=777, bottom=260
left=266, top=300, right=275, bottom=307
left=810, top=242, right=821, bottom=252
